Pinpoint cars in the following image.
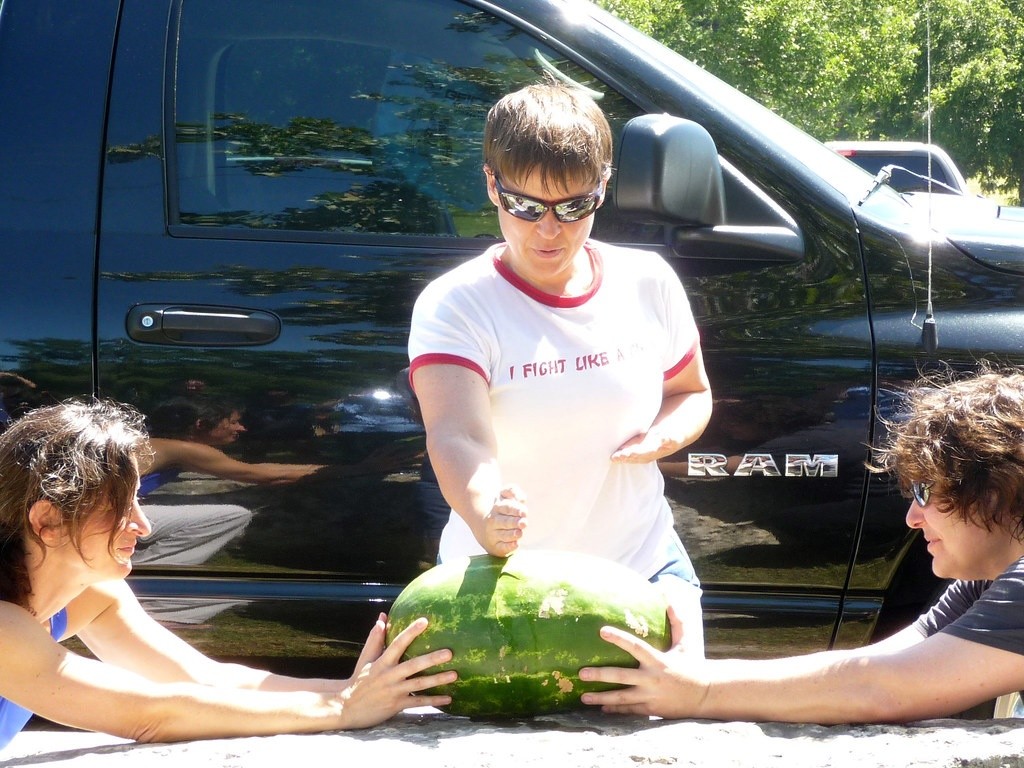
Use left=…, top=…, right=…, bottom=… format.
left=823, top=138, right=973, bottom=202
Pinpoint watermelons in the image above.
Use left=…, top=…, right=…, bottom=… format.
left=385, top=550, right=674, bottom=717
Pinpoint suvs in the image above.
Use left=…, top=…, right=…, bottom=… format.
left=0, top=1, right=1023, bottom=679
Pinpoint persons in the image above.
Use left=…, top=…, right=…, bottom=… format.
left=0, top=399, right=459, bottom=762
left=579, top=369, right=1024, bottom=723
left=407, top=82, right=715, bottom=664
left=658, top=391, right=915, bottom=559
left=129, top=401, right=330, bottom=568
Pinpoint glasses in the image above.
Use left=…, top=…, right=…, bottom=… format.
left=909, top=481, right=936, bottom=508
left=494, top=177, right=604, bottom=223
